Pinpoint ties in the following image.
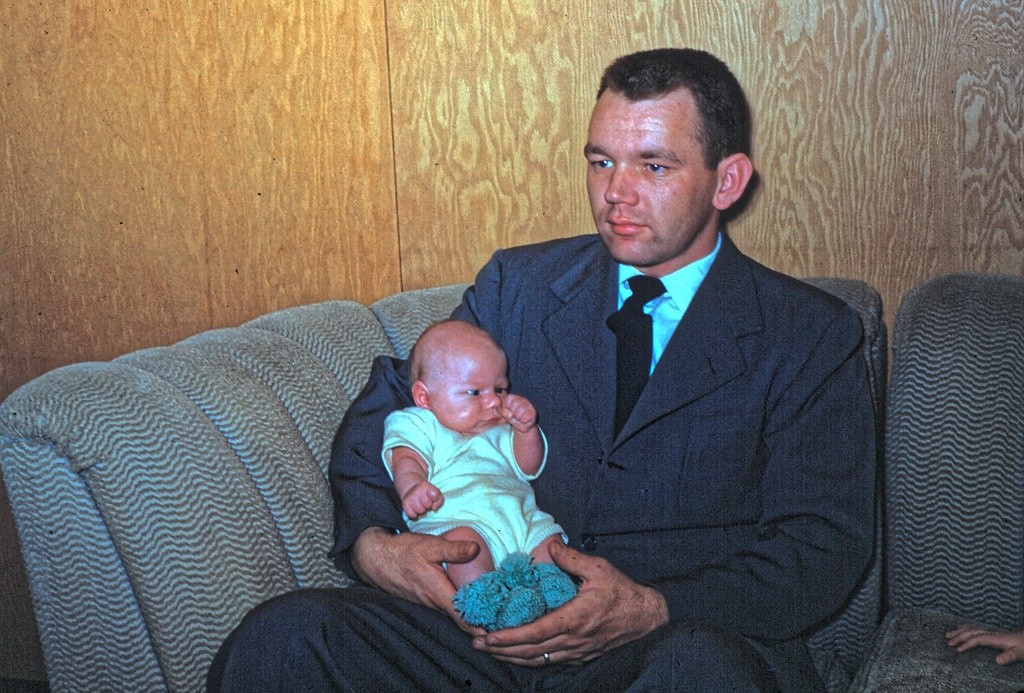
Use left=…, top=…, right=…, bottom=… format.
left=607, top=274, right=669, bottom=443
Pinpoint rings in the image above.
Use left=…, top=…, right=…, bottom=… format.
left=543, top=653, right=550, bottom=665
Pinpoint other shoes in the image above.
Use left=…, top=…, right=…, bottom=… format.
left=451, top=571, right=546, bottom=630
left=501, top=552, right=577, bottom=609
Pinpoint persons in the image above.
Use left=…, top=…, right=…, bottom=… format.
left=945, top=624, right=1023, bottom=665
left=382, top=319, right=578, bottom=633
left=208, top=49, right=882, bottom=693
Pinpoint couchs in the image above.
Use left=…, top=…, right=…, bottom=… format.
left=2, top=269, right=1023, bottom=692
